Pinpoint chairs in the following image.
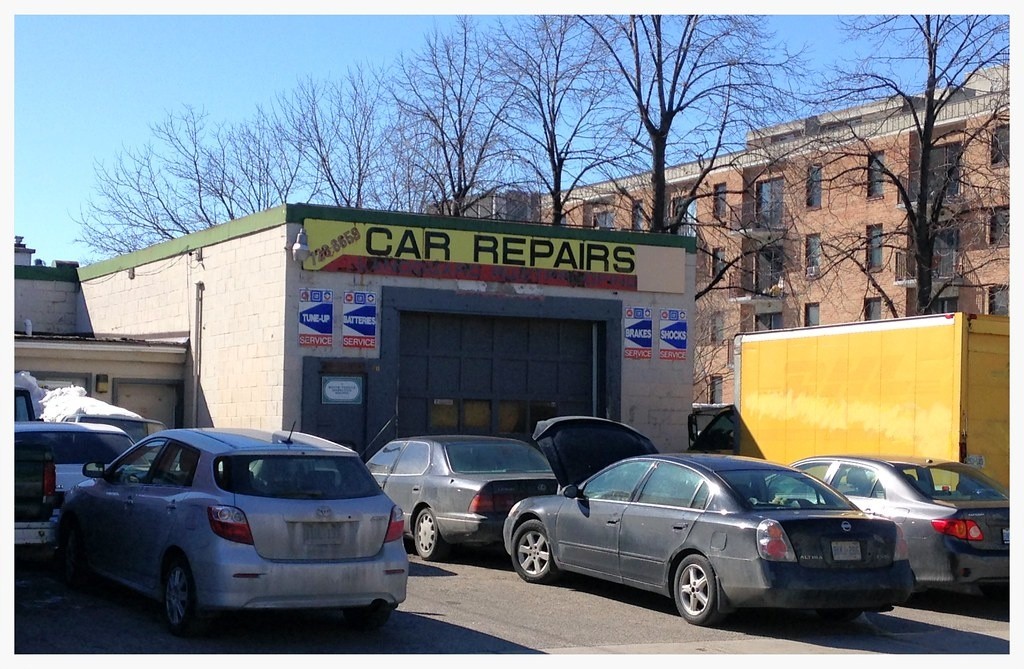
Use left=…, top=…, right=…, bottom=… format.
left=845, top=466, right=877, bottom=497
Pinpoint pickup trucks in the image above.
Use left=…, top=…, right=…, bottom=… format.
left=14, top=386, right=57, bottom=574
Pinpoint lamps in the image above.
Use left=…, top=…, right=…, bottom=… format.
left=291, top=226, right=308, bottom=260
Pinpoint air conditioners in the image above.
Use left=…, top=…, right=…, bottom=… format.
left=807, top=265, right=819, bottom=276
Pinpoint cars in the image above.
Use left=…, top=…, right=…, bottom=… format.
left=15, top=422, right=153, bottom=523
left=355, top=435, right=560, bottom=560
left=762, top=455, right=1010, bottom=605
left=58, top=426, right=410, bottom=635
left=61, top=415, right=181, bottom=466
left=503, top=416, right=913, bottom=627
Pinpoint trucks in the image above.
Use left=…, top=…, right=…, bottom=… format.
left=688, top=313, right=1010, bottom=496
left=693, top=403, right=733, bottom=432
